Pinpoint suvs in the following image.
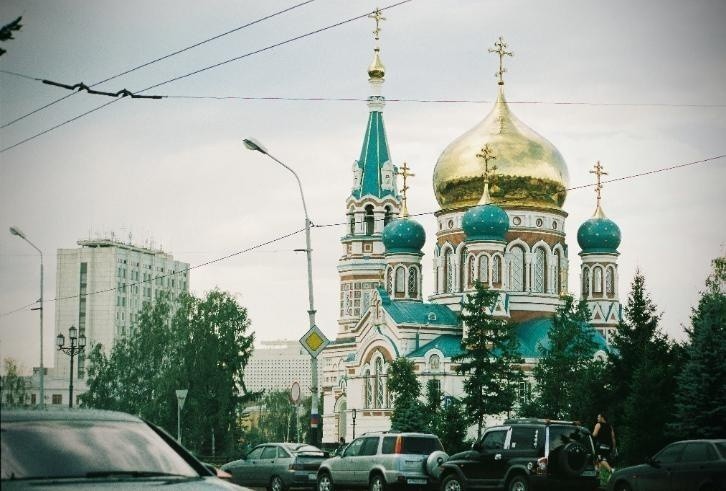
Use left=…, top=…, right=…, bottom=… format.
left=438, top=419, right=601, bottom=491
left=316, top=431, right=451, bottom=491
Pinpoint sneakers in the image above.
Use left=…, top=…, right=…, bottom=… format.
left=607, top=468, right=615, bottom=483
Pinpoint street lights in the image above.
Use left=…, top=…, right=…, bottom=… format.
left=8, top=225, right=45, bottom=407
left=242, top=135, right=322, bottom=447
left=57, top=326, right=87, bottom=409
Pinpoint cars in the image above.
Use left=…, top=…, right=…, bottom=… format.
left=608, top=439, right=726, bottom=491
left=0, top=408, right=253, bottom=491
left=220, top=442, right=330, bottom=491
